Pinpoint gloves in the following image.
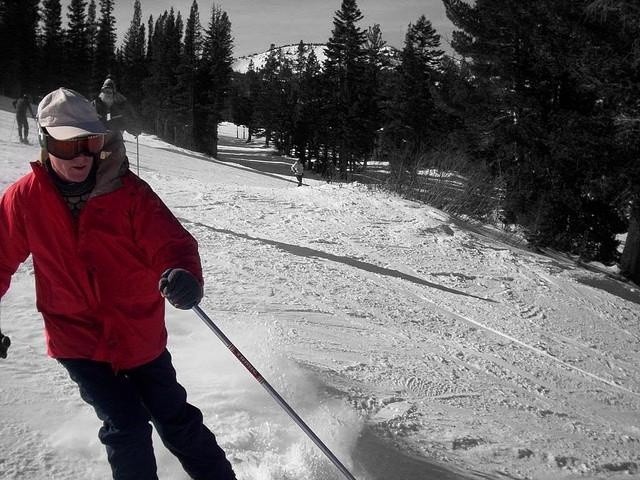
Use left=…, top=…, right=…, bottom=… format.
left=158, top=268, right=203, bottom=310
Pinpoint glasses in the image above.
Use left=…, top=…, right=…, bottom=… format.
left=41, top=127, right=104, bottom=160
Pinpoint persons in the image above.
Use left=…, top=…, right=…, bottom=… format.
left=12, top=92, right=36, bottom=143
left=291, top=157, right=304, bottom=187
left=0, top=87, right=236, bottom=479
left=90, top=74, right=140, bottom=139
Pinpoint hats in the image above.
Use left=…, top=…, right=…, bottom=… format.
left=36, top=87, right=106, bottom=141
